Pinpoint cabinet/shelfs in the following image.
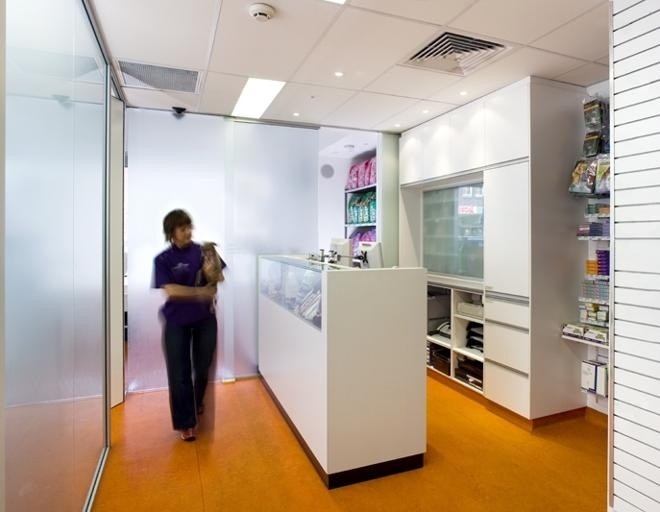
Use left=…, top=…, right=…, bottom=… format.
left=398, top=75, right=588, bottom=432
left=255, top=252, right=430, bottom=491
left=318, top=127, right=399, bottom=266
left=559, top=186, right=611, bottom=350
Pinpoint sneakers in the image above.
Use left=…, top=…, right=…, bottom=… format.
left=197, top=407, right=203, bottom=416
left=181, top=428, right=195, bottom=441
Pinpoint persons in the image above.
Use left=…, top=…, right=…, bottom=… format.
left=154, top=210, right=226, bottom=440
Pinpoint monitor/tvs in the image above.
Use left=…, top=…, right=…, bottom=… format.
left=359, top=241, right=383, bottom=267
left=329, top=238, right=352, bottom=266
